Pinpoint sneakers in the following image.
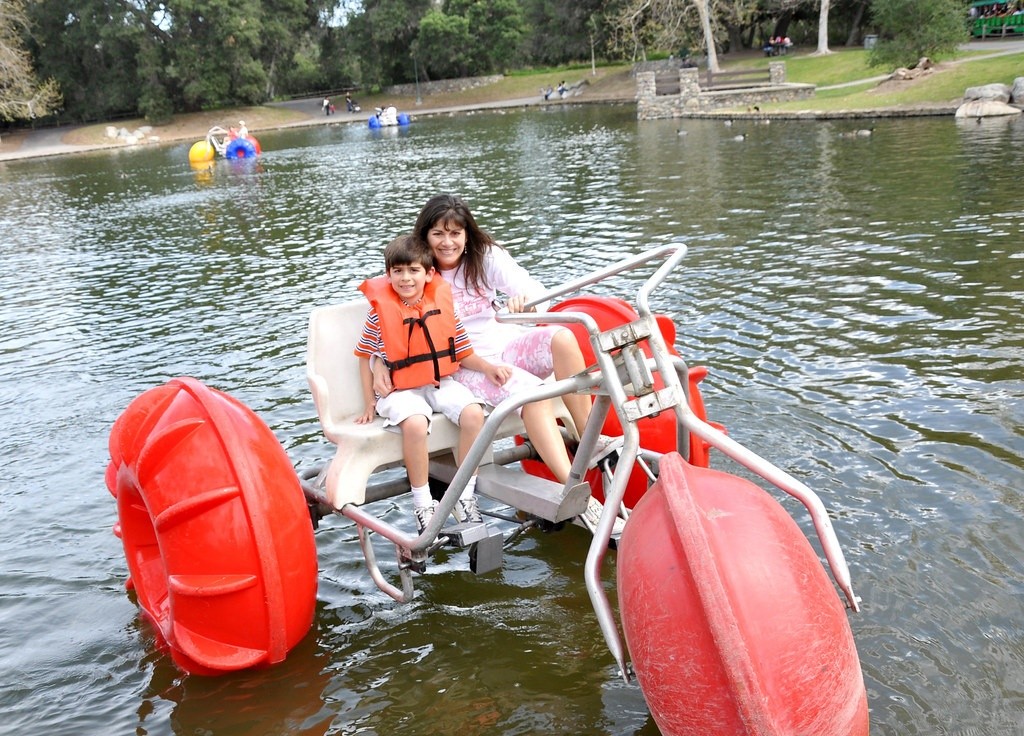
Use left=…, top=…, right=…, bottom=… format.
left=588, top=434, right=625, bottom=471
left=450, top=496, right=484, bottom=524
left=577, top=495, right=627, bottom=538
left=413, top=499, right=440, bottom=546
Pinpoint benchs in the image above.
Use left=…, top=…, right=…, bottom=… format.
left=306, top=298, right=581, bottom=511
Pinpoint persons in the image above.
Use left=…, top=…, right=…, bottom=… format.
left=369, top=195, right=643, bottom=538
left=353, top=235, right=511, bottom=543
left=544, top=85, right=552, bottom=100
left=227, top=120, right=248, bottom=140
left=322, top=96, right=335, bottom=115
left=345, top=92, right=353, bottom=114
left=763, top=35, right=790, bottom=57
left=557, top=80, right=568, bottom=95
left=968, top=2, right=1024, bottom=19
